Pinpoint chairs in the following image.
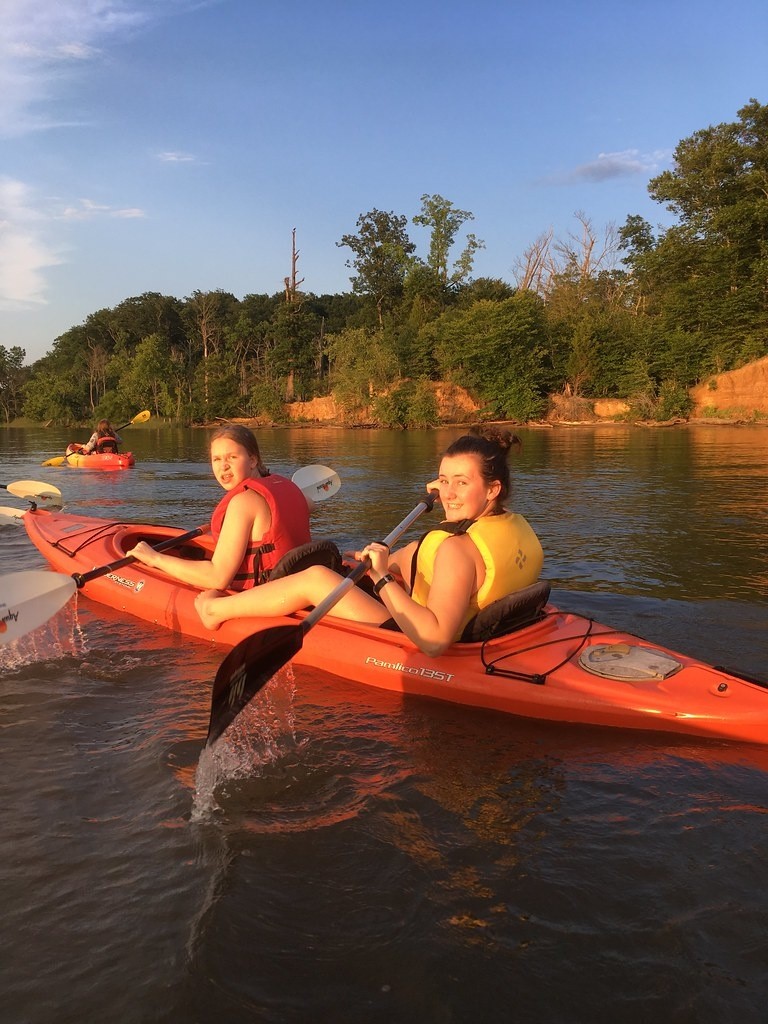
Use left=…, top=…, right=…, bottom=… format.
left=266, top=539, right=342, bottom=602
left=460, top=579, right=552, bottom=646
left=97, top=436, right=118, bottom=456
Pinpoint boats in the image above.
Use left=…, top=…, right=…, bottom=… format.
left=21, top=501, right=768, bottom=745
left=65, top=443, right=136, bottom=466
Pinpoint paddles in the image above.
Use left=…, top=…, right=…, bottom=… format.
left=40, top=409, right=152, bottom=466
left=0, top=479, right=63, bottom=506
left=0, top=461, right=344, bottom=651
left=203, top=488, right=441, bottom=749
left=0, top=506, right=28, bottom=527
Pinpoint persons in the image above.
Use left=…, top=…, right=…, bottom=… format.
left=127, top=424, right=314, bottom=592
left=82, top=419, right=123, bottom=455
left=193, top=421, right=546, bottom=659
